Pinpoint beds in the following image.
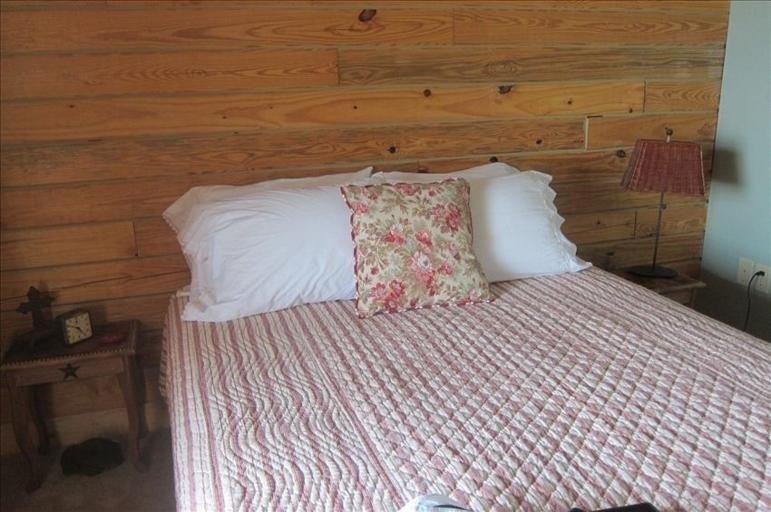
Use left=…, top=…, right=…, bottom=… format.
left=163, top=266, right=770, bottom=512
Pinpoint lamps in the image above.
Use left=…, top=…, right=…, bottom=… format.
left=617, top=127, right=707, bottom=279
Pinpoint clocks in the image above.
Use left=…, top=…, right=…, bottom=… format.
left=54, top=307, right=95, bottom=347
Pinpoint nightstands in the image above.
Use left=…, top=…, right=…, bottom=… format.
left=609, top=268, right=709, bottom=309
left=0, top=318, right=150, bottom=494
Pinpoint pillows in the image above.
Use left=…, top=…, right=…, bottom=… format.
left=339, top=176, right=496, bottom=321
left=164, top=165, right=375, bottom=322
left=447, top=161, right=521, bottom=177
left=371, top=169, right=594, bottom=282
